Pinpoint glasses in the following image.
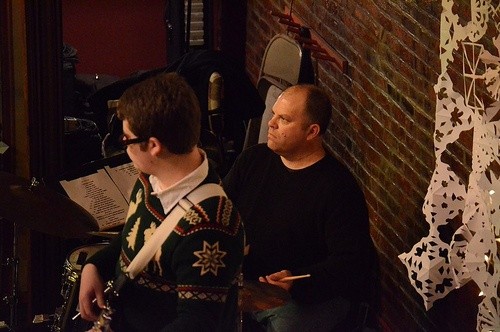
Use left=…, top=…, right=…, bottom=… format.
left=119, top=134, right=149, bottom=150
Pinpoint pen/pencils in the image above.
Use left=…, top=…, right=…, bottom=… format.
left=280, top=274, right=310, bottom=281
left=72, top=287, right=110, bottom=320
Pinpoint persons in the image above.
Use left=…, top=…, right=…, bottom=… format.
left=78, top=72, right=246, bottom=332
left=220, top=83, right=374, bottom=332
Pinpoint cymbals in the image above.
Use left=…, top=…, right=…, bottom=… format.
left=237, top=280, right=289, bottom=311
left=0, top=185, right=100, bottom=238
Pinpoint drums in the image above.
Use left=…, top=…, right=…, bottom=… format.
left=60, top=242, right=113, bottom=332
